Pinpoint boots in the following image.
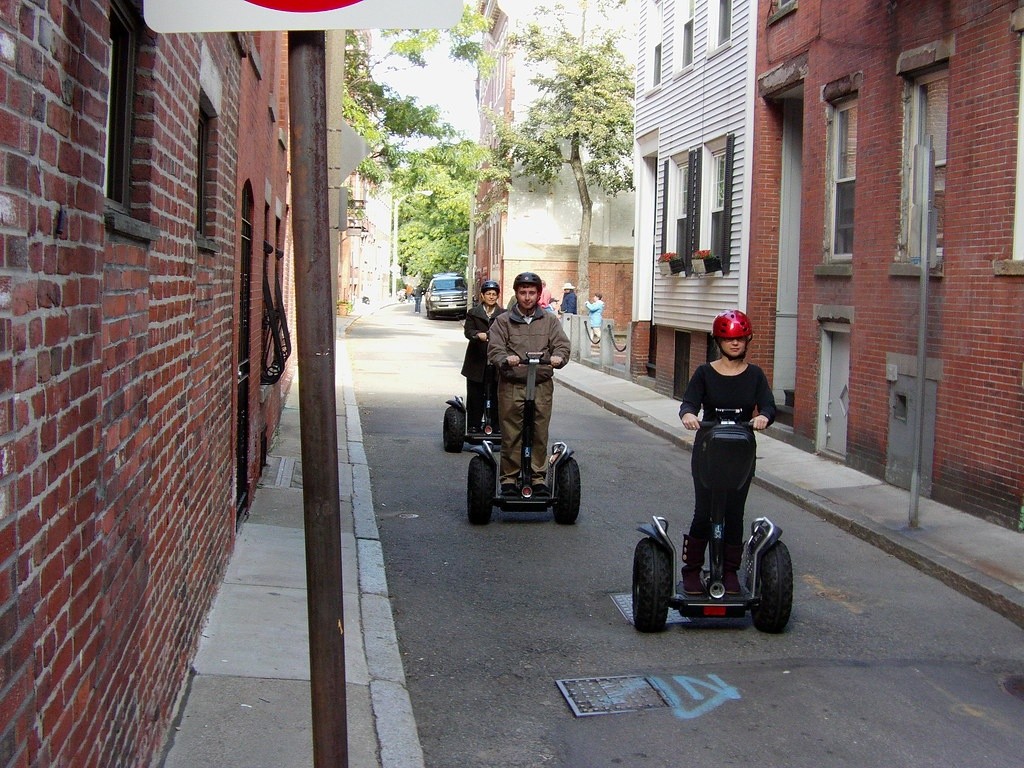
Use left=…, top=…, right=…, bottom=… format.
left=681, top=534, right=708, bottom=595
left=722, top=540, right=747, bottom=595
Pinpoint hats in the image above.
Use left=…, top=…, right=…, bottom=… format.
left=563, top=283, right=575, bottom=290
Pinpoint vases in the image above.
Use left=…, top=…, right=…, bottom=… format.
left=658, top=257, right=686, bottom=276
left=691, top=256, right=722, bottom=274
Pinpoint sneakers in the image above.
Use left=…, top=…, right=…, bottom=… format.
left=500, top=483, right=518, bottom=495
left=532, top=483, right=549, bottom=495
left=469, top=424, right=479, bottom=432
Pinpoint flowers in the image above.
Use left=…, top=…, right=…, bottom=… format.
left=658, top=252, right=680, bottom=264
left=691, top=249, right=715, bottom=260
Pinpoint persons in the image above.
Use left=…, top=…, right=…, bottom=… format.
left=508, top=280, right=578, bottom=320
left=461, top=280, right=508, bottom=434
left=405, top=283, right=413, bottom=303
left=415, top=284, right=426, bottom=313
left=585, top=293, right=605, bottom=348
left=679, top=309, right=778, bottom=594
left=487, top=272, right=571, bottom=496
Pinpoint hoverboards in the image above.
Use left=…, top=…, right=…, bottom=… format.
left=466, top=353, right=582, bottom=525
left=442, top=332, right=503, bottom=454
left=633, top=418, right=793, bottom=637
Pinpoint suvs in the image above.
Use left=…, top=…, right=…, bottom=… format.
left=424, top=273, right=468, bottom=320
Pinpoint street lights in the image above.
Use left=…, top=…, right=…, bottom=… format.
left=392, top=190, right=433, bottom=297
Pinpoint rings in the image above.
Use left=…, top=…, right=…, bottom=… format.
left=692, top=426, right=696, bottom=428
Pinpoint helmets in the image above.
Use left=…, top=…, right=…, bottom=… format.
left=513, top=272, right=542, bottom=289
left=713, top=309, right=752, bottom=338
left=481, top=281, right=500, bottom=290
